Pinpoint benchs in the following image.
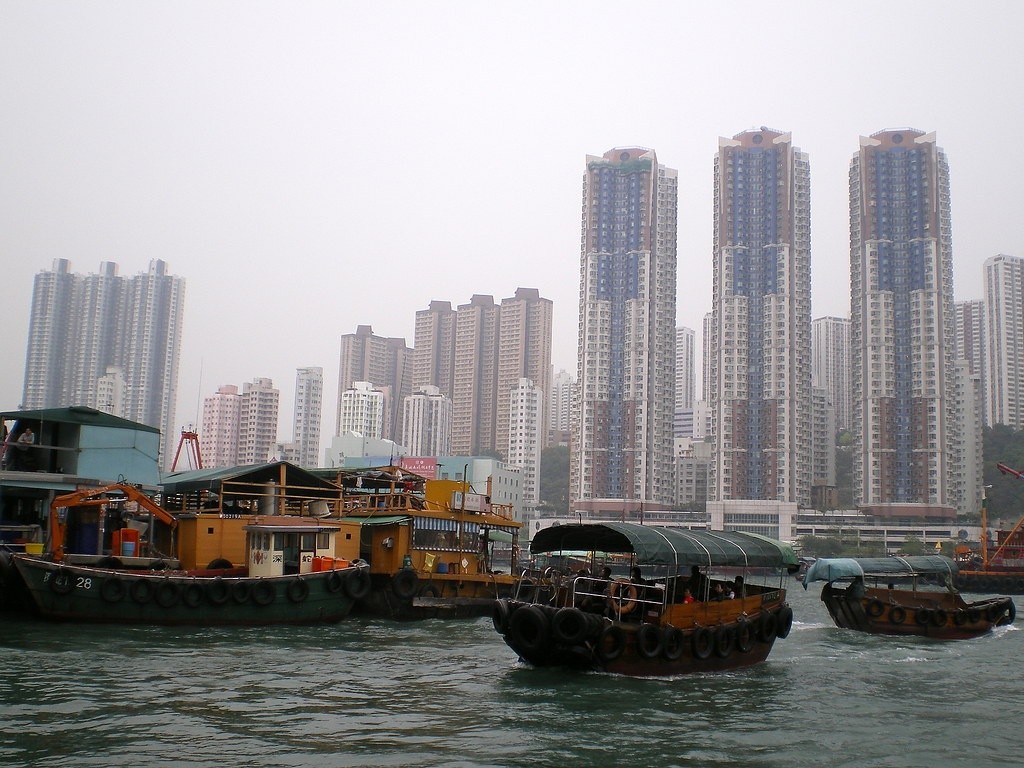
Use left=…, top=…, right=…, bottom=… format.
left=630, top=576, right=786, bottom=628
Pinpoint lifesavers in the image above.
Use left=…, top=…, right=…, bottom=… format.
left=636, top=624, right=685, bottom=662
left=888, top=606, right=906, bottom=625
left=985, top=604, right=999, bottom=623
left=49, top=568, right=77, bottom=597
left=610, top=578, right=638, bottom=615
left=953, top=609, right=981, bottom=626
left=417, top=584, right=440, bottom=598
left=342, top=570, right=372, bottom=602
left=979, top=577, right=1024, bottom=592
left=866, top=599, right=884, bottom=618
left=491, top=598, right=627, bottom=663
left=391, top=569, right=420, bottom=599
left=690, top=606, right=793, bottom=661
left=914, top=607, right=947, bottom=627
left=206, top=558, right=234, bottom=570
left=99, top=575, right=276, bottom=606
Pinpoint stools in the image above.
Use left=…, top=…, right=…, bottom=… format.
left=448, top=563, right=459, bottom=574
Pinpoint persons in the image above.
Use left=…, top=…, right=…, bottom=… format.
left=888, top=583, right=894, bottom=590
left=847, top=576, right=865, bottom=594
left=1, top=416, right=9, bottom=441
left=526, top=562, right=748, bottom=624
left=16, top=428, right=35, bottom=445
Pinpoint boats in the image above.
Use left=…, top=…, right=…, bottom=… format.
left=1, top=461, right=378, bottom=632
left=802, top=555, right=1016, bottom=641
left=306, top=357, right=523, bottom=619
left=489, top=517, right=801, bottom=675
left=957, top=485, right=1024, bottom=595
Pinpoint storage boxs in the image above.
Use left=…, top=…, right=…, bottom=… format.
left=112, top=528, right=139, bottom=557
left=25, top=543, right=44, bottom=554
